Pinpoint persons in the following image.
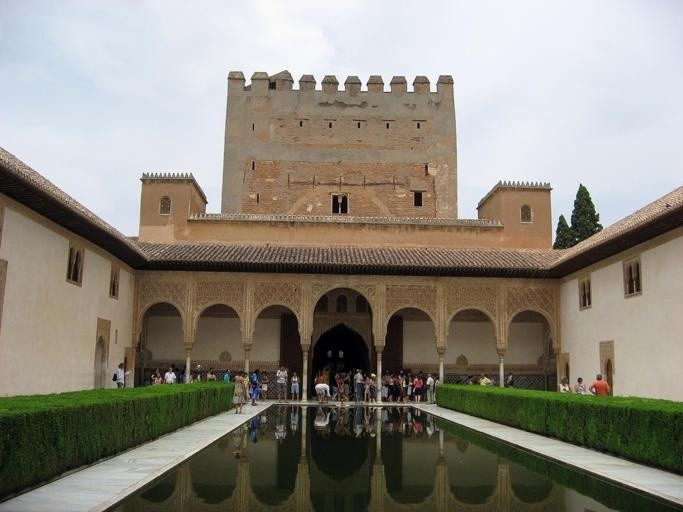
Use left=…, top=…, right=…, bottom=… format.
left=116, top=362, right=131, bottom=389
left=469, top=371, right=515, bottom=388
left=560, top=374, right=611, bottom=396
left=233, top=407, right=436, bottom=459
left=144, top=364, right=439, bottom=406
left=229, top=370, right=248, bottom=414
